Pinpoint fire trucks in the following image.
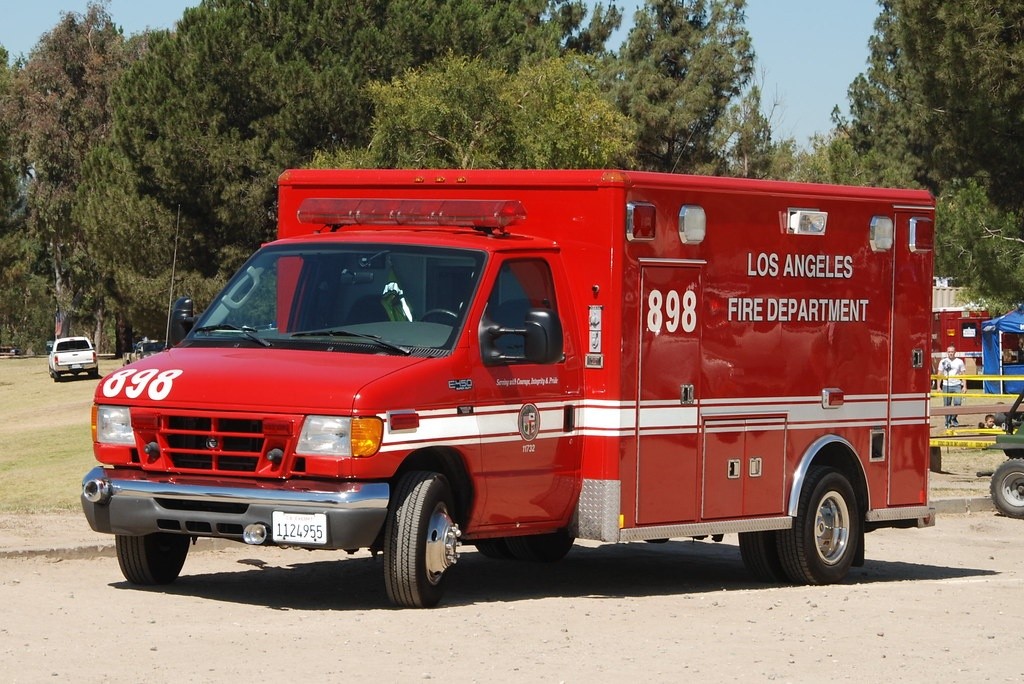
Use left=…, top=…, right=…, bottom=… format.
left=933, top=304, right=1024, bottom=390
left=80, top=168, right=935, bottom=605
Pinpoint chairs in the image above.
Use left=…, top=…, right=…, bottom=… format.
left=322, top=265, right=389, bottom=325
left=492, top=299, right=550, bottom=357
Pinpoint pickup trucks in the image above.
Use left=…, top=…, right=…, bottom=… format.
left=46, top=336, right=99, bottom=382
left=122, top=340, right=173, bottom=366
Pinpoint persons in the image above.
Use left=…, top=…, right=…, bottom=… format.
left=978, top=415, right=995, bottom=429
left=937, top=346, right=967, bottom=428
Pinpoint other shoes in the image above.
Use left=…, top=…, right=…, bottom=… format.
left=944, top=418, right=960, bottom=429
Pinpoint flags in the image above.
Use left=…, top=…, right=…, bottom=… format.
left=55, top=308, right=62, bottom=336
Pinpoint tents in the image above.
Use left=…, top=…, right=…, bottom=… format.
left=982, top=304, right=1024, bottom=394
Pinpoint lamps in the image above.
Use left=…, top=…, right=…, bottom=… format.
left=296, top=196, right=530, bottom=238
left=790, top=208, right=828, bottom=236
